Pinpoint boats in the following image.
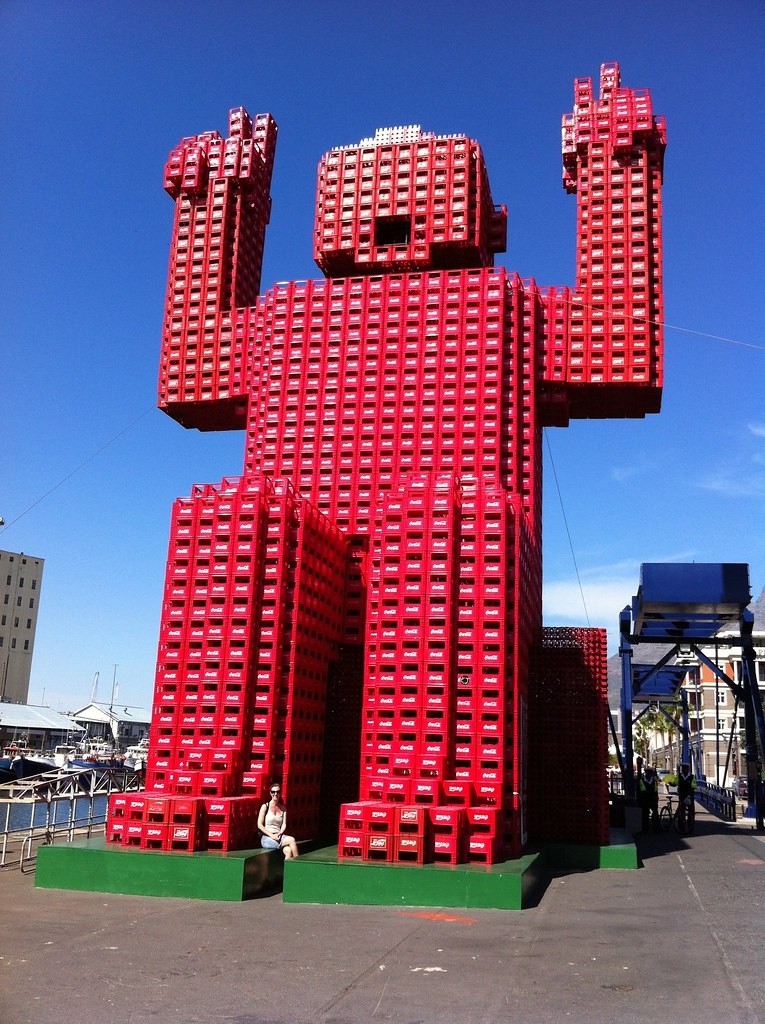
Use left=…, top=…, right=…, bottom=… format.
left=0, top=735, right=149, bottom=791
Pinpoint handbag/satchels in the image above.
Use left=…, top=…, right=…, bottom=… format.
left=257, top=802, right=269, bottom=839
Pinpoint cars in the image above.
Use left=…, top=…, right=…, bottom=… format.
left=732, top=776, right=748, bottom=799
left=657, top=770, right=671, bottom=782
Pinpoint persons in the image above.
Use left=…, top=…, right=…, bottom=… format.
left=668, top=763, right=697, bottom=834
left=638, top=767, right=660, bottom=833
left=257, top=784, right=299, bottom=860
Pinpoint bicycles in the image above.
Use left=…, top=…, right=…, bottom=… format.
left=660, top=796, right=693, bottom=836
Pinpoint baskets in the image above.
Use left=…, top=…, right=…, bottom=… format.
left=679, top=791, right=694, bottom=806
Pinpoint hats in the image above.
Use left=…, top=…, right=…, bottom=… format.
left=680, top=763, right=690, bottom=767
left=644, top=768, right=653, bottom=771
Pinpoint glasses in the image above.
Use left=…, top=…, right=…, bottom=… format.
left=270, top=790, right=280, bottom=794
